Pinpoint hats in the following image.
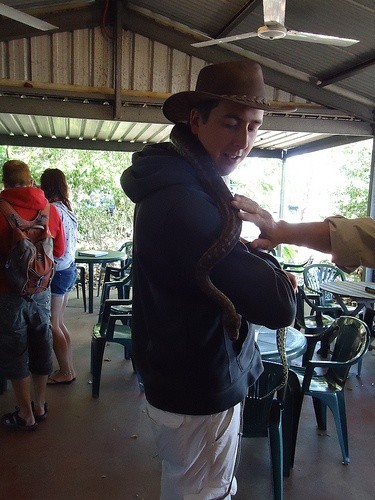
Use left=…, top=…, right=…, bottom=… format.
left=161, top=57, right=299, bottom=126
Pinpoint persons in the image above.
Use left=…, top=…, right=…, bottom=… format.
left=0, top=160, right=54, bottom=432
left=231, top=193, right=375, bottom=275
left=120, top=60, right=298, bottom=500
left=40, top=169, right=79, bottom=386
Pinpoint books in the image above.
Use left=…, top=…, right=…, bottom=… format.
left=78, top=250, right=108, bottom=257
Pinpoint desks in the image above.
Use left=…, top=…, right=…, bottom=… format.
left=75, top=249, right=128, bottom=313
left=319, top=282, right=375, bottom=316
left=255, top=324, right=308, bottom=363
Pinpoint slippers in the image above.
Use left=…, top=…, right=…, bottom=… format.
left=0, top=396, right=50, bottom=433
left=46, top=369, right=78, bottom=386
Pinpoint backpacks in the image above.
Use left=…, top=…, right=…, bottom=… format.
left=0, top=195, right=56, bottom=300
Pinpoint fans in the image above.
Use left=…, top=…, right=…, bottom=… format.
left=191, top=0, right=361, bottom=47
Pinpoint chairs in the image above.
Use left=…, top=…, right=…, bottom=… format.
left=72, top=242, right=375, bottom=500
